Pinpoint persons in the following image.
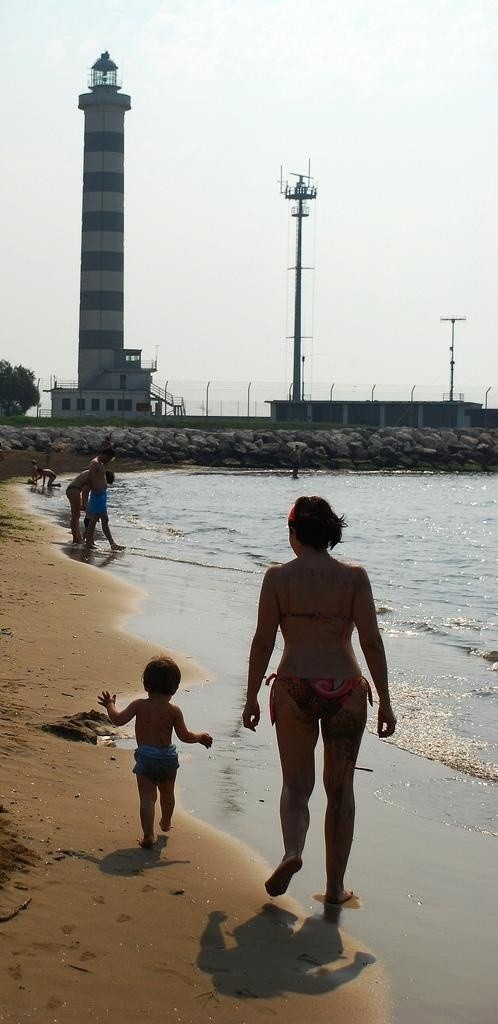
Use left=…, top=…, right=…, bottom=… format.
left=31, top=460, right=38, bottom=484
left=97, top=657, right=213, bottom=848
left=289, top=445, right=299, bottom=479
left=243, top=497, right=396, bottom=904
left=34, top=468, right=61, bottom=487
left=66, top=448, right=126, bottom=550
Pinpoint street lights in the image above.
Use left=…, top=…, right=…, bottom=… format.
left=441, top=318, right=466, bottom=401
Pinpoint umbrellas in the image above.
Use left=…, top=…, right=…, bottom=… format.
left=286, top=442, right=307, bottom=449
